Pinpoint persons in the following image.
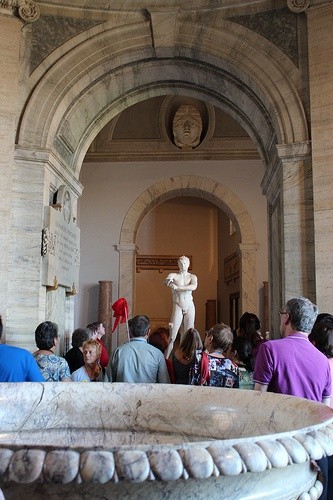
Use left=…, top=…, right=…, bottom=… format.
left=172, top=104, right=202, bottom=147
left=163, top=255, right=198, bottom=358
left=190, top=296, right=333, bottom=500
left=171, top=328, right=203, bottom=384
left=0, top=313, right=173, bottom=384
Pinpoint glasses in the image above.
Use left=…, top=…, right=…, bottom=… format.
left=279, top=312, right=287, bottom=317
left=205, top=330, right=209, bottom=337
